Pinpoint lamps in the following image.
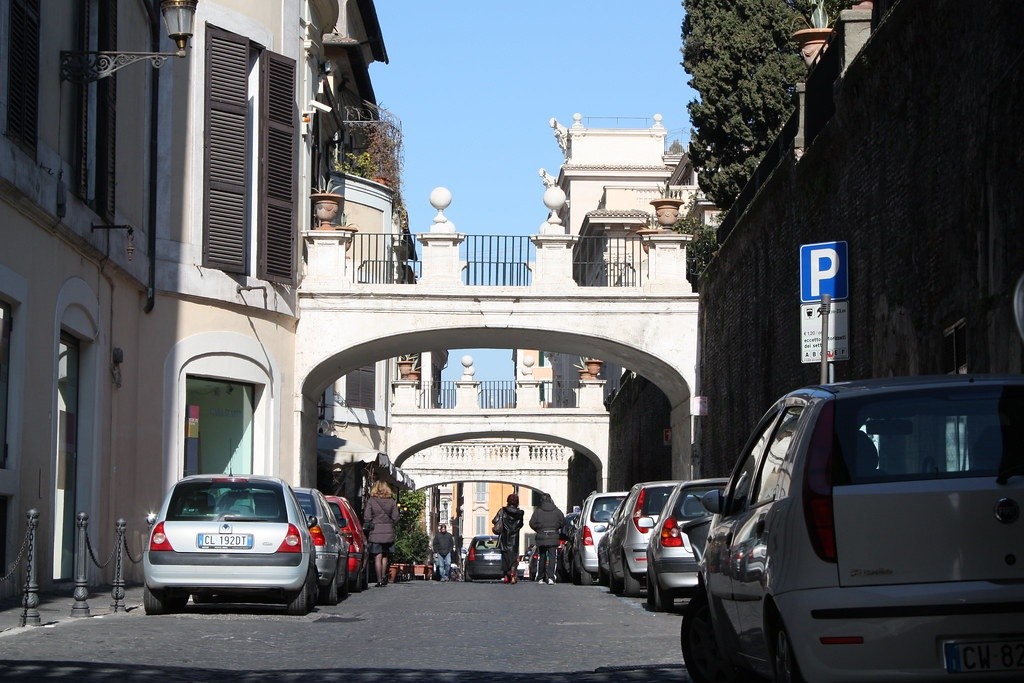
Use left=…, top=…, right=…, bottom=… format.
left=60, top=0, right=199, bottom=84
left=318, top=56, right=331, bottom=75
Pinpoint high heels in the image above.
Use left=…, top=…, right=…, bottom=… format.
left=382, top=581, right=387, bottom=587
left=375, top=582, right=382, bottom=588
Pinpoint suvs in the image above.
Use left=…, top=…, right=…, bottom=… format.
left=559, top=489, right=629, bottom=587
left=682, top=376, right=1024, bottom=683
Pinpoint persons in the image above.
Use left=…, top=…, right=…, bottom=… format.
left=433, top=523, right=454, bottom=582
left=433, top=544, right=465, bottom=581
left=492, top=494, right=524, bottom=584
left=529, top=493, right=566, bottom=585
left=364, top=480, right=399, bottom=588
left=480, top=541, right=489, bottom=548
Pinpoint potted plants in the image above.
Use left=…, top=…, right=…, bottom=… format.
left=308, top=175, right=344, bottom=230
left=395, top=352, right=419, bottom=372
left=371, top=161, right=387, bottom=185
left=635, top=212, right=664, bottom=254
left=405, top=359, right=421, bottom=380
left=364, top=484, right=433, bottom=583
left=692, top=229, right=704, bottom=243
left=783, top=0, right=848, bottom=70
left=401, top=369, right=413, bottom=380
left=584, top=356, right=604, bottom=377
left=649, top=180, right=685, bottom=229
left=572, top=356, right=600, bottom=380
left=334, top=210, right=358, bottom=259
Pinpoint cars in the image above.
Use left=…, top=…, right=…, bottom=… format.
left=518, top=545, right=558, bottom=584
left=593, top=478, right=687, bottom=595
left=323, top=496, right=368, bottom=592
left=140, top=474, right=319, bottom=616
left=638, top=476, right=731, bottom=609
left=212, top=487, right=347, bottom=605
left=463, top=535, right=505, bottom=583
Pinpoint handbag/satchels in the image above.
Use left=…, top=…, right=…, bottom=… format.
left=492, top=508, right=504, bottom=535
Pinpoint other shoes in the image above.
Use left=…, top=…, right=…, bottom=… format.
left=548, top=578, right=557, bottom=585
left=538, top=579, right=547, bottom=584
left=504, top=577, right=510, bottom=583
left=511, top=576, right=516, bottom=583
left=441, top=578, right=445, bottom=582
left=445, top=576, right=449, bottom=581
